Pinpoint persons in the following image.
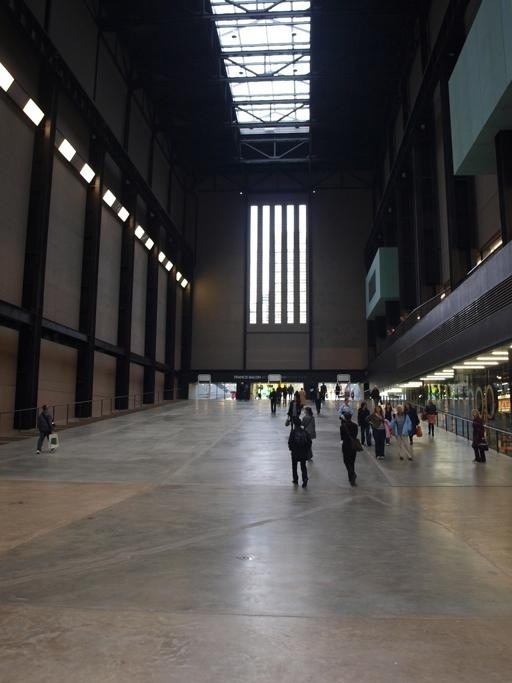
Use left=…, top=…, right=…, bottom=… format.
left=363, top=405, right=386, bottom=461
left=338, top=411, right=359, bottom=486
left=288, top=416, right=312, bottom=488
left=470, top=408, right=487, bottom=464
left=36, top=404, right=58, bottom=454
left=388, top=404, right=413, bottom=460
left=301, top=404, right=318, bottom=463
left=256, top=386, right=262, bottom=399
left=268, top=378, right=438, bottom=446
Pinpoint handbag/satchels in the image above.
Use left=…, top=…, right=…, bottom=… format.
left=48, top=420, right=55, bottom=428
left=285, top=416, right=290, bottom=426
left=416, top=426, right=422, bottom=437
left=353, top=438, right=363, bottom=451
left=421, top=414, right=427, bottom=421
left=478, top=440, right=488, bottom=451
left=48, top=433, right=58, bottom=448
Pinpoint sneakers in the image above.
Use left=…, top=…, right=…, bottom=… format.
left=293, top=478, right=308, bottom=487
left=377, top=456, right=384, bottom=460
left=368, top=443, right=371, bottom=447
left=37, top=450, right=40, bottom=454
left=350, top=472, right=356, bottom=485
left=473, top=458, right=485, bottom=463
